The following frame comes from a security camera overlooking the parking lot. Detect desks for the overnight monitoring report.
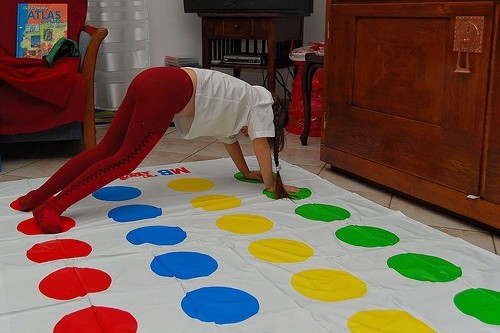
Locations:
[198,10,311,95]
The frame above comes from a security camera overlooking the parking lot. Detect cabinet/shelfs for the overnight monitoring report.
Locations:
[320,0,495,230]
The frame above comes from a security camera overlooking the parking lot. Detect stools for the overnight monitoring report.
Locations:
[299,52,324,146]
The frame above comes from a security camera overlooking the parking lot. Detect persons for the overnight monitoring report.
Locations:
[19,66,301,234]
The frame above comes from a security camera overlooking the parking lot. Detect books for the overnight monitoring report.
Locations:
[164,55,199,67]
[16,2,68,59]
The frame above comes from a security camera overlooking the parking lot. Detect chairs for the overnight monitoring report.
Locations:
[0,0,108,151]
